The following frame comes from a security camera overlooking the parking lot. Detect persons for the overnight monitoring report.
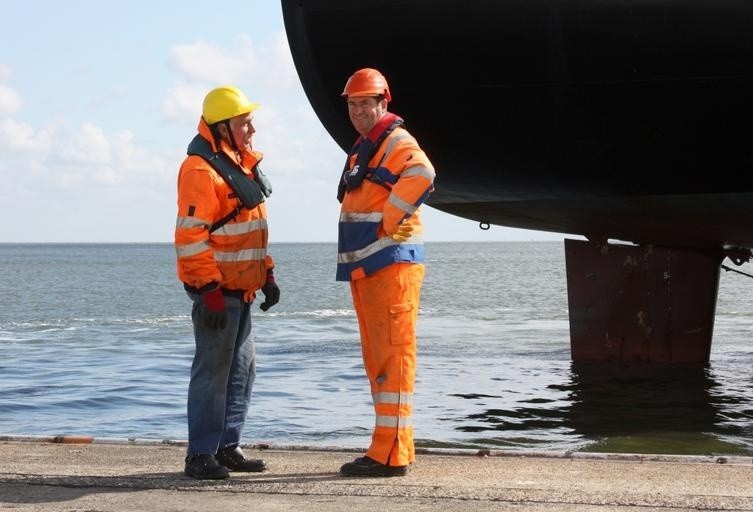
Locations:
[331,66,441,478]
[172,85,280,480]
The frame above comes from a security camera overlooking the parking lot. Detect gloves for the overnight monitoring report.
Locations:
[260,271,281,311]
[387,219,414,243]
[201,284,228,330]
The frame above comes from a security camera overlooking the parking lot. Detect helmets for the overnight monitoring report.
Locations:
[202,85,259,124]
[340,66,392,103]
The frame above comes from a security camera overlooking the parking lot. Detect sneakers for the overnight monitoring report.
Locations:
[354,455,413,472]
[340,463,408,476]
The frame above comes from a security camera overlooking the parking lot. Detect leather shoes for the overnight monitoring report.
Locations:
[216,447,269,473]
[185,454,230,481]
